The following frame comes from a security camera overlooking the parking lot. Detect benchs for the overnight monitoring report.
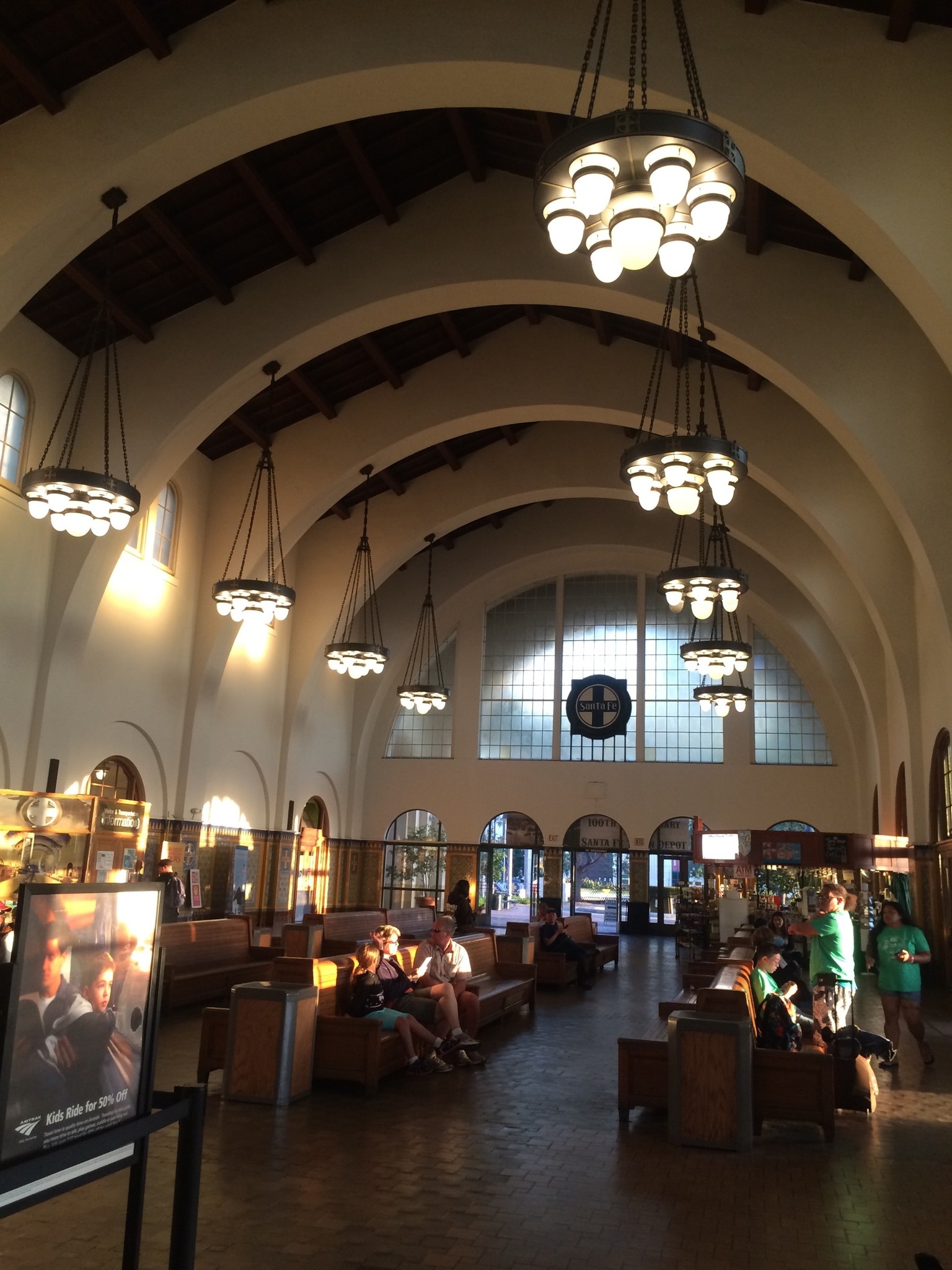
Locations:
[502,899,522,908]
[159,905,837,1146]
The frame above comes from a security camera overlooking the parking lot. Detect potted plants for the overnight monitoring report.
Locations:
[385,823,447,907]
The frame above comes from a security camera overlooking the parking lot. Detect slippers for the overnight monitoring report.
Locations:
[918,1041,934,1065]
[879,1060,899,1070]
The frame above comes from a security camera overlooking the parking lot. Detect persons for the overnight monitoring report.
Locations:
[4,921,137,1124]
[413,915,487,1068]
[541,907,593,989]
[343,942,461,1076]
[445,879,484,935]
[372,925,480,1067]
[750,911,815,1051]
[157,858,179,923]
[0,901,15,963]
[787,882,858,1032]
[865,901,935,1070]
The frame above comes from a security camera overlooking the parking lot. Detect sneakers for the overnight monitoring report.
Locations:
[404,1058,432,1074]
[467,1049,487,1065]
[425,1056,452,1072]
[435,1037,461,1058]
[445,1032,479,1045]
[455,1049,471,1066]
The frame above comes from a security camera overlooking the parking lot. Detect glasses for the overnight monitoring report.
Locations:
[430,927,447,933]
[816,894,844,902]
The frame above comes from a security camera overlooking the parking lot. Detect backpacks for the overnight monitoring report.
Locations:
[164,874,186,908]
[755,993,802,1052]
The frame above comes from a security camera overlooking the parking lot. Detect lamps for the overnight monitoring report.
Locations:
[22,186,146,538]
[190,808,201,819]
[656,493,749,621]
[679,600,753,681]
[396,534,451,715]
[532,0,747,285]
[94,764,110,780]
[211,360,296,626]
[693,672,753,717]
[324,462,389,679]
[619,264,750,517]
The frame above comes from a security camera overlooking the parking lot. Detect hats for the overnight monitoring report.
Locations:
[546,907,556,914]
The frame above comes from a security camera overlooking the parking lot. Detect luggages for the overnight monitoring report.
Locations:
[826,979,879,1119]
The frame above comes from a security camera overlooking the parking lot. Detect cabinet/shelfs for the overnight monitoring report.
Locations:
[675,897,711,964]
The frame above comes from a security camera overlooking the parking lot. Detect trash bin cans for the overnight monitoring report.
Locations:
[496,935,535,964]
[252,926,272,947]
[282,923,323,958]
[664,1008,753,1148]
[221,979,319,1107]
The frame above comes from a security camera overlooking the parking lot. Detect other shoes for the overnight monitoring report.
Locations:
[582,988,596,998]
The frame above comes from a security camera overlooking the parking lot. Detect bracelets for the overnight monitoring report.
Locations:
[910,954,914,963]
